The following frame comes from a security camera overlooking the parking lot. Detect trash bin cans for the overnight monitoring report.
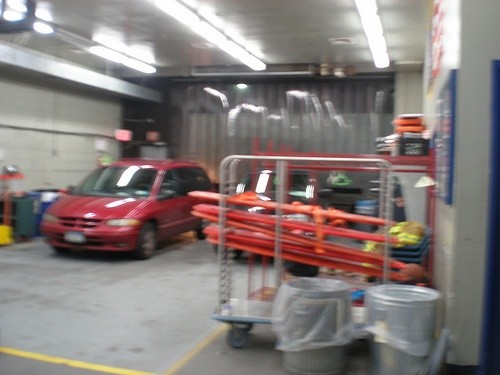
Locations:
[368,283,442,375]
[280,277,350,375]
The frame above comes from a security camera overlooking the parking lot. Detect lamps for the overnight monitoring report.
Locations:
[355,0,391,69]
[149,0,267,71]
[87,44,156,75]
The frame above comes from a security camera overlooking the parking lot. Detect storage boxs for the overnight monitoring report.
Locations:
[395,137,431,156]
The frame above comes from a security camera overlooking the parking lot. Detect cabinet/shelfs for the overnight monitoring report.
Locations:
[0,196,35,237]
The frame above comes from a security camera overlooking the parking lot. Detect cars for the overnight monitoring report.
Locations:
[39,158,219,260]
[212,167,338,262]
